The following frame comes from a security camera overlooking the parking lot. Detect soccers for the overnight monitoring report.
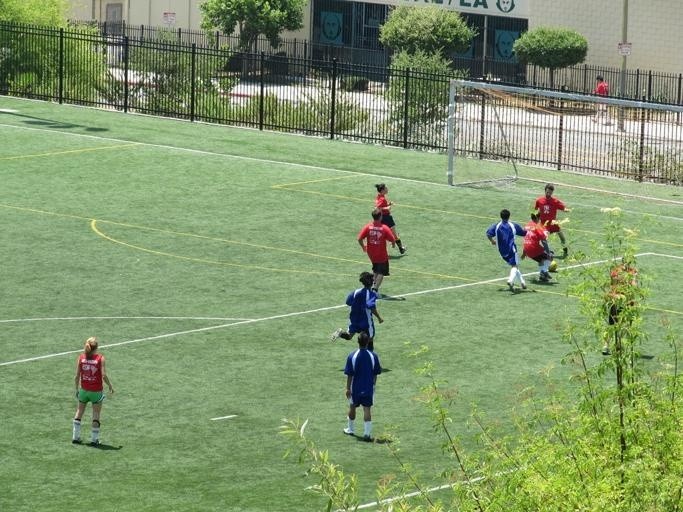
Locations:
[548,261,557,272]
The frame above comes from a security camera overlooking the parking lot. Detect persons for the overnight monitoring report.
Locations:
[592,75,611,125]
[602,258,639,356]
[344,333,381,441]
[72,338,115,447]
[330,273,384,352]
[375,184,407,255]
[486,210,527,292]
[520,211,554,282]
[358,208,396,298]
[535,184,571,257]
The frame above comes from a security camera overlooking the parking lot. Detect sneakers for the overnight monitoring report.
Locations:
[329,328,343,344]
[71,437,102,446]
[505,270,553,291]
[600,346,613,356]
[371,287,381,299]
[398,244,408,255]
[341,427,374,441]
